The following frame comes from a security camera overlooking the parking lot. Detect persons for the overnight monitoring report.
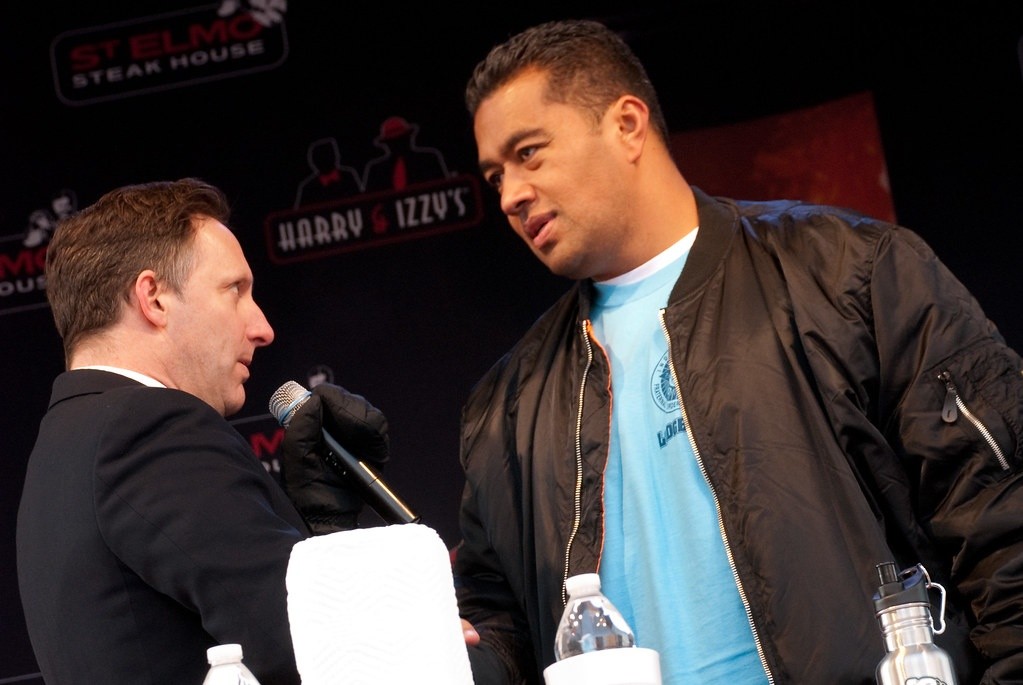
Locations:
[16,176,479,685]
[451,22,1023,685]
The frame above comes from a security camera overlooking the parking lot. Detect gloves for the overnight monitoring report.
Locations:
[279,383,393,538]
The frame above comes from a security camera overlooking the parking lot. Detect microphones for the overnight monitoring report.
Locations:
[269,381,424,525]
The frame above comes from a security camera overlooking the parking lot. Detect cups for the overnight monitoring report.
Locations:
[542,646,663,685]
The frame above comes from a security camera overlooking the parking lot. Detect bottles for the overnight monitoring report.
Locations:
[554,573,635,663]
[201,643,261,685]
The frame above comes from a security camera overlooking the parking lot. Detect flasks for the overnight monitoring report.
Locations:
[872,560,958,685]
